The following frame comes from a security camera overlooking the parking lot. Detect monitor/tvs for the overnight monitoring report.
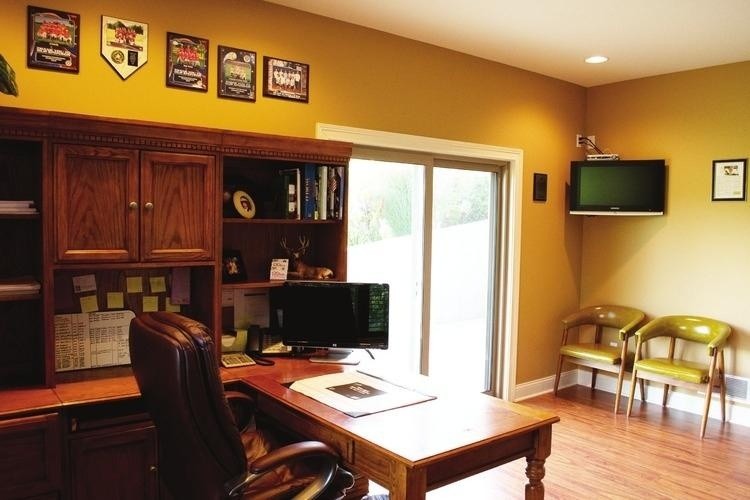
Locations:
[568,160,665,216]
[269,283,389,364]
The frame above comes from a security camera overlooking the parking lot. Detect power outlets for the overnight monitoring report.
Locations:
[586,135,595,149]
[576,134,583,148]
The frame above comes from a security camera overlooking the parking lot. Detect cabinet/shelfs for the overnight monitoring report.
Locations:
[0,105,358,499]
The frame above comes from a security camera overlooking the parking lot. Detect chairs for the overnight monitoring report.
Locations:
[553,304,647,413]
[626,315,732,439]
[128,310,354,500]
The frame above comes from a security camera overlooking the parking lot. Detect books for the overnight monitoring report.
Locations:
[279,162,346,222]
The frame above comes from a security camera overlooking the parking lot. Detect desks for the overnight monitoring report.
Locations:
[242,362,560,499]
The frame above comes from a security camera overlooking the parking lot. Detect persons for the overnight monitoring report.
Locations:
[241,197,251,212]
[175,43,202,70]
[229,64,248,82]
[114,24,137,46]
[37,19,73,44]
[275,66,300,91]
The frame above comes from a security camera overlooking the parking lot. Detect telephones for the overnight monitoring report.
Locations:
[248,324,293,353]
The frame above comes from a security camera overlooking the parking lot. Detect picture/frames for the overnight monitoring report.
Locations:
[712,159,747,201]
[101,15,148,80]
[217,44,256,102]
[26,5,80,74]
[263,55,310,104]
[165,31,209,93]
